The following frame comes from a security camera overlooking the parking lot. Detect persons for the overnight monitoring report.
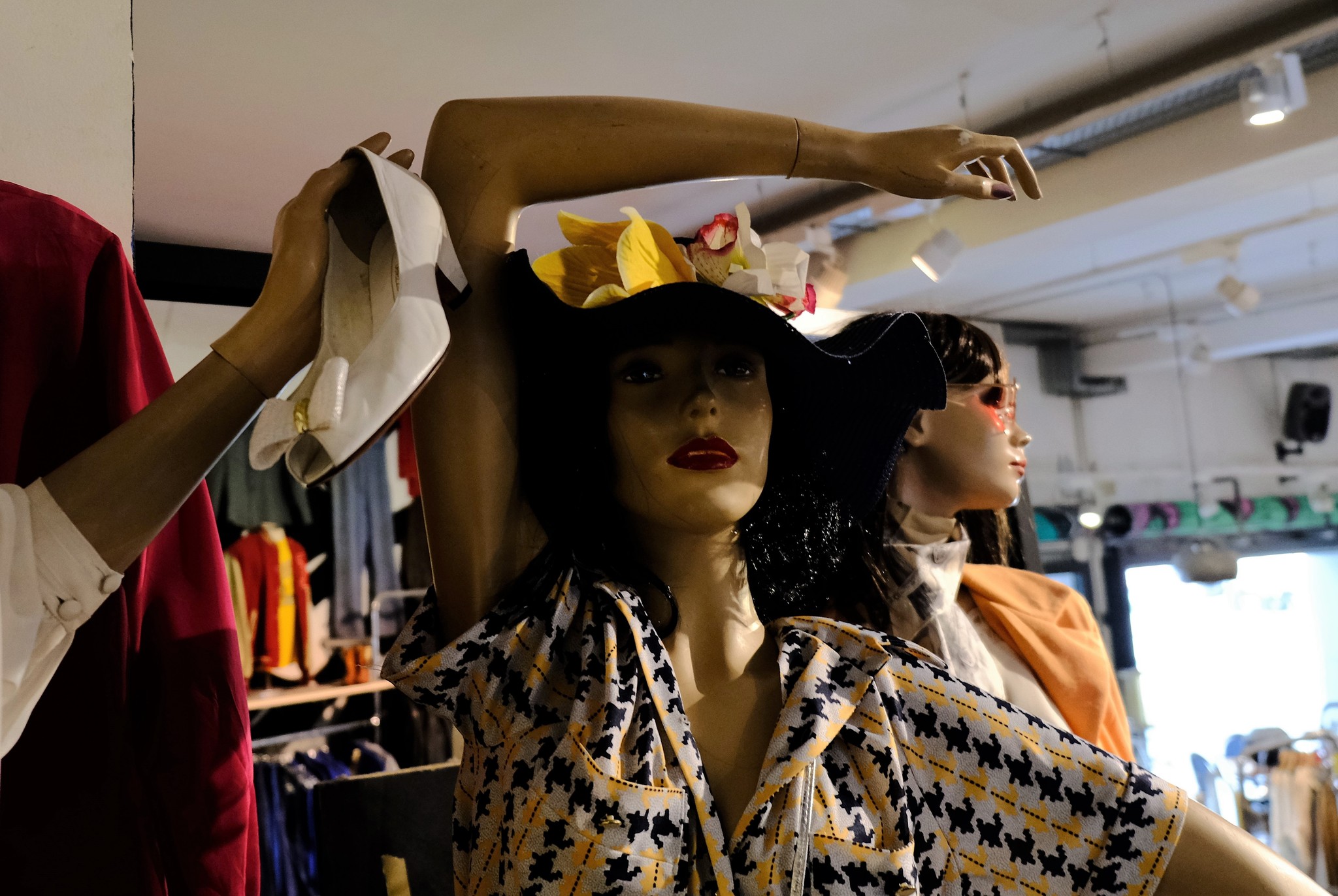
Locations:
[0,130,410,765]
[375,96,1332,896]
[812,310,1137,767]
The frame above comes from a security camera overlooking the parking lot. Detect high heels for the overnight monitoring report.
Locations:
[251,148,471,491]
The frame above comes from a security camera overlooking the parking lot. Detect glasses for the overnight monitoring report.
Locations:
[948,383,1017,431]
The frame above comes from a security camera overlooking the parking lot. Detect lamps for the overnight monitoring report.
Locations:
[1233,59,1303,146]
[1074,492,1109,537]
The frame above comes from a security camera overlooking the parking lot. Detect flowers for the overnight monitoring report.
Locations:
[534,203,828,327]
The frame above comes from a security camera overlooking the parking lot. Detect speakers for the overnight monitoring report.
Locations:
[1284,382,1331,442]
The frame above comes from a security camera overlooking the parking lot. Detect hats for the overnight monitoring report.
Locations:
[509,241,950,617]
